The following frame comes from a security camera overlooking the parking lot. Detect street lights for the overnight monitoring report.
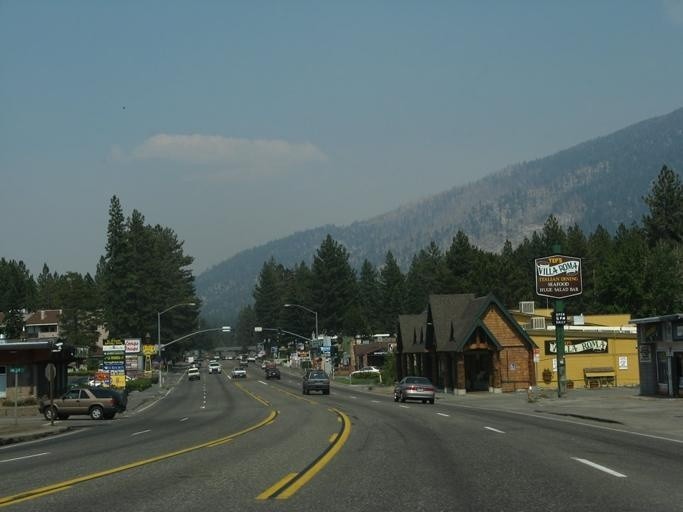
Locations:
[158,303,196,387]
[284,304,318,338]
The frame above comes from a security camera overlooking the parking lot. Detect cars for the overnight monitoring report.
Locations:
[262,361,280,380]
[352,366,379,375]
[231,367,246,378]
[394,376,435,404]
[303,370,329,395]
[187,362,201,380]
[40,388,127,419]
[240,358,255,367]
[208,360,223,374]
